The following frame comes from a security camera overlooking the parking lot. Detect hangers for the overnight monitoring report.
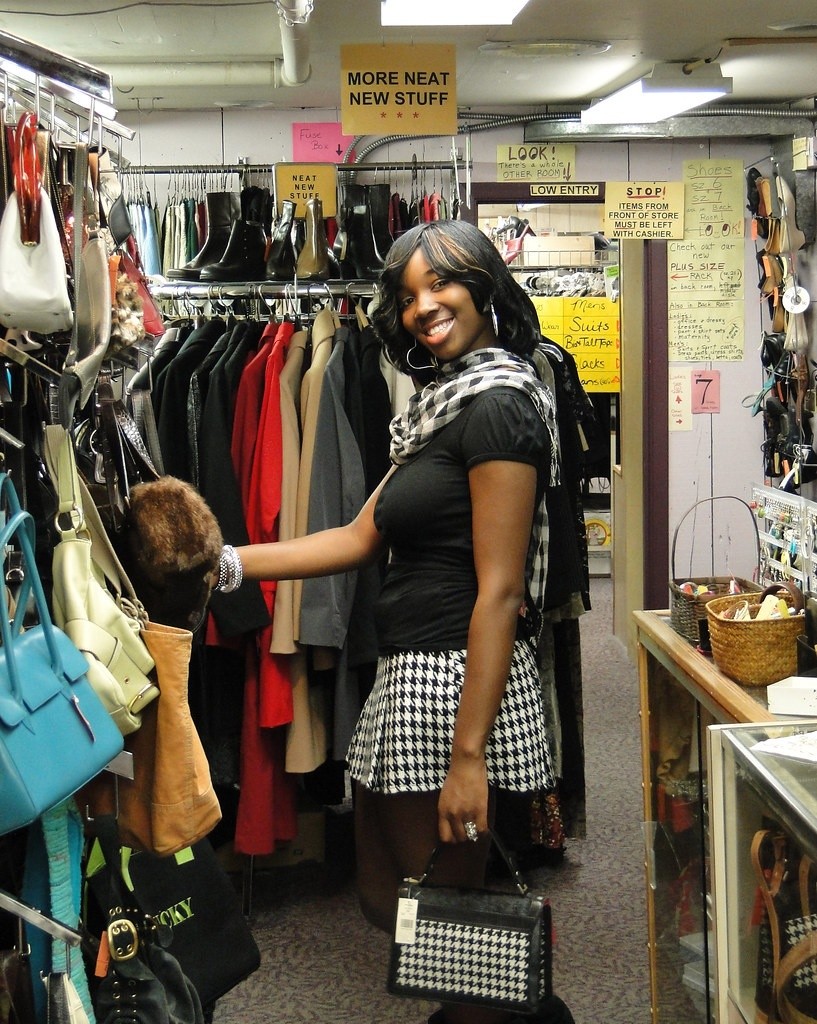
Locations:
[523,272,607,298]
[112,160,457,212]
[152,283,383,334]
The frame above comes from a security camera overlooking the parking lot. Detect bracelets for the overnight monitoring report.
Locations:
[212,545,242,593]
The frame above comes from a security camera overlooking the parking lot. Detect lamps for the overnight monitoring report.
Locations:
[579,59,732,125]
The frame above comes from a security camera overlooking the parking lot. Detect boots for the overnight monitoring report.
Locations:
[167,183,401,283]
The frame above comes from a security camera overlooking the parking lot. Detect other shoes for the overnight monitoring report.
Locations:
[743,166,817,498]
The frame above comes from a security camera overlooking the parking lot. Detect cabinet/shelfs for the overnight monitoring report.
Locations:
[633,609,817,1023]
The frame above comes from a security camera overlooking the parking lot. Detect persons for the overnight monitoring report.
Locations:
[211,219,573,1024]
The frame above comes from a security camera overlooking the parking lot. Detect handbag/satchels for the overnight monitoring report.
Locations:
[0,101,261,1024]
[748,810,817,1024]
[384,826,553,1014]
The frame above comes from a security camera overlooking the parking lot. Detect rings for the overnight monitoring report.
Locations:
[463,822,478,842]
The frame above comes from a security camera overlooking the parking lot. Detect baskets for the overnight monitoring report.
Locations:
[668,495,761,645]
[704,592,806,687]
